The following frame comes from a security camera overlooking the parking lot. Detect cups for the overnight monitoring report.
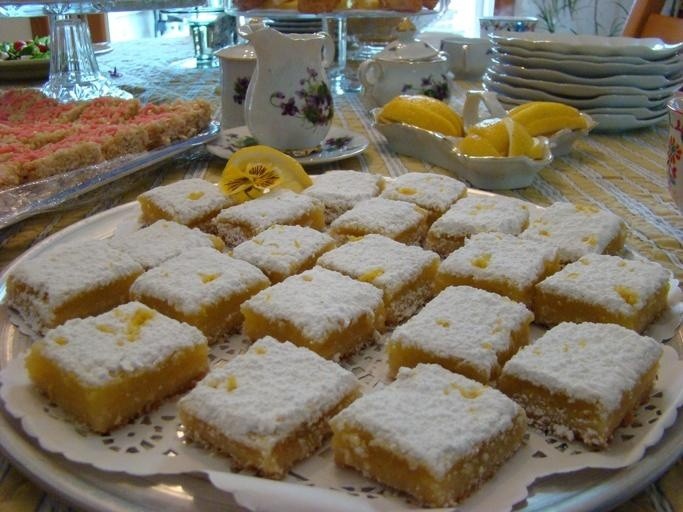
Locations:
[215,41,257,119]
[442,37,490,77]
[479,17,538,40]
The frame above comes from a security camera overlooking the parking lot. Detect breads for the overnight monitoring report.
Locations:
[519,201,625,261]
[110,217,215,267]
[329,362,528,505]
[379,171,467,217]
[498,322,663,448]
[329,197,432,247]
[136,176,228,228]
[176,336,364,480]
[317,233,441,330]
[228,222,336,284]
[533,251,671,338]
[240,265,388,362]
[6,237,145,335]
[129,243,272,346]
[433,231,560,304]
[384,285,533,385]
[25,300,211,434]
[423,196,530,257]
[215,190,325,245]
[1,88,210,194]
[299,170,386,225]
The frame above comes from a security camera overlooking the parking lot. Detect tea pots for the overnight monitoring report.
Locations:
[358,17,452,105]
[237,24,334,151]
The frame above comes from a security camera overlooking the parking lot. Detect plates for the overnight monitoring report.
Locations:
[482,83,670,118]
[490,40,683,64]
[491,48,683,78]
[483,72,683,109]
[487,32,683,61]
[269,22,322,27]
[371,108,594,189]
[501,102,670,120]
[277,27,322,33]
[0,41,113,74]
[590,113,669,131]
[0,184,683,512]
[488,67,683,100]
[265,13,323,21]
[205,125,370,164]
[492,58,683,89]
